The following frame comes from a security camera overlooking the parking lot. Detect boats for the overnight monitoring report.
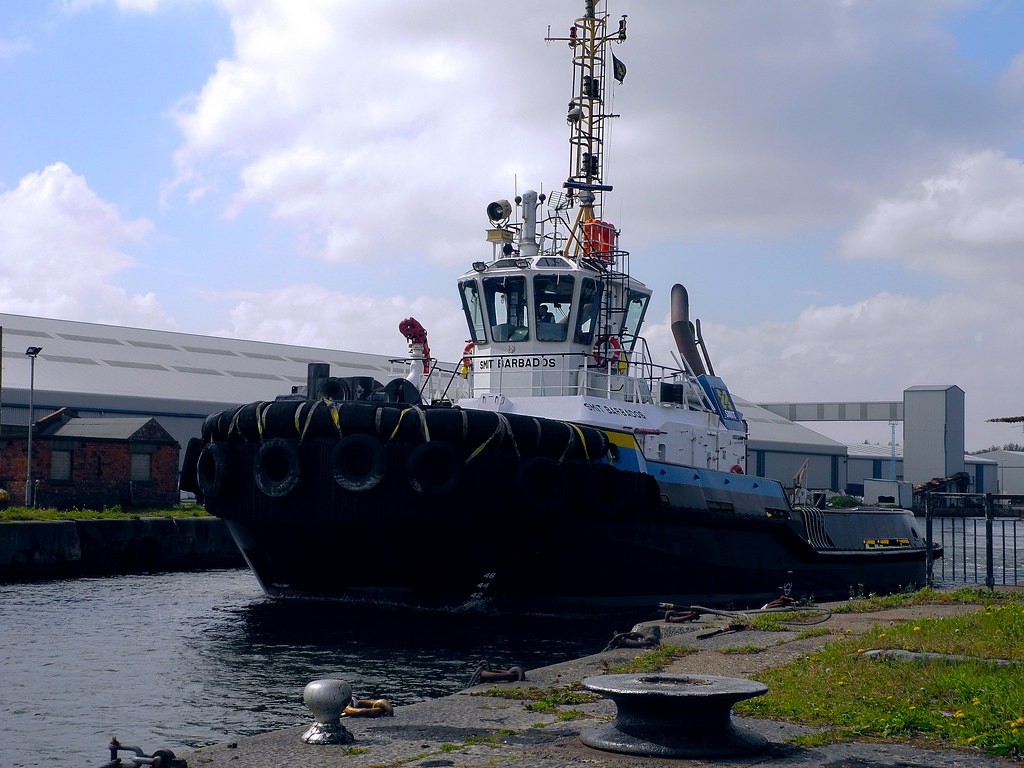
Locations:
[178,2,825,637]
[791,504,943,599]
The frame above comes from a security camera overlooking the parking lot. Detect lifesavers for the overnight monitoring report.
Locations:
[462,343,474,364]
[516,454,566,512]
[330,434,388,493]
[592,336,620,366]
[582,462,628,520]
[195,443,231,499]
[251,438,300,498]
[406,441,462,497]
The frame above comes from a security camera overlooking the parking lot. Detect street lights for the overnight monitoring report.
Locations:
[25,347,42,507]
[999,459,1007,507]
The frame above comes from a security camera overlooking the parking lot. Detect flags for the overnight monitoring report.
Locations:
[612,53,626,84]
[794,458,807,486]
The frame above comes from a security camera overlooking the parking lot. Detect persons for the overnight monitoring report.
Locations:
[538,304,555,322]
